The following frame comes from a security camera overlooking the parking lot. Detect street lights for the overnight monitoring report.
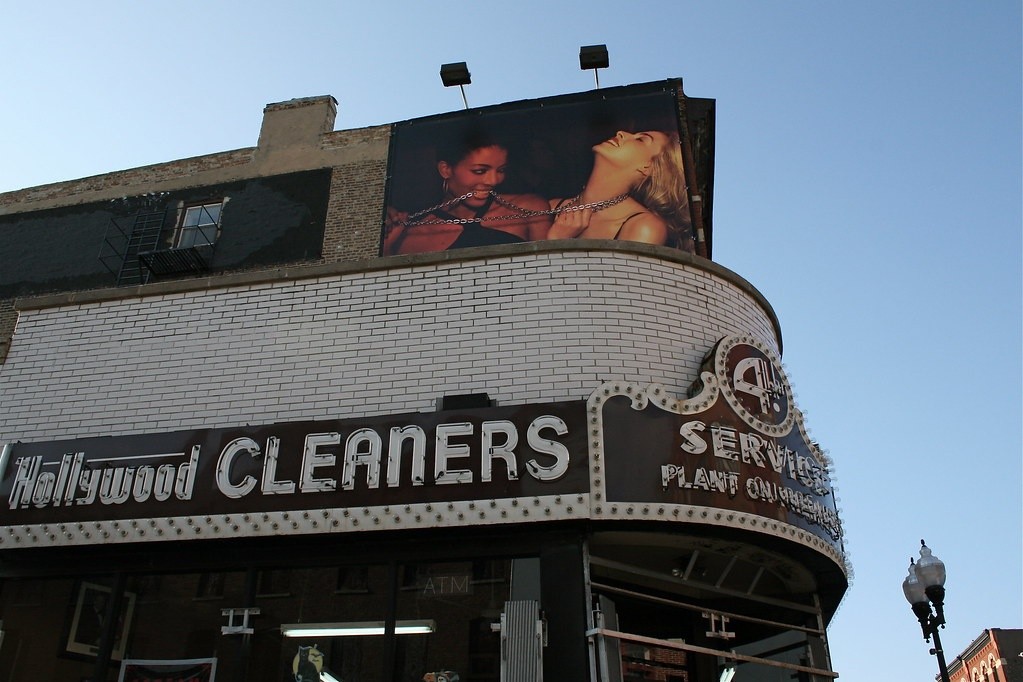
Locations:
[902,539,951,682]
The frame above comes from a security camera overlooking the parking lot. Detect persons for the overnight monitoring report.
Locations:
[548,131,694,253]
[384,135,552,255]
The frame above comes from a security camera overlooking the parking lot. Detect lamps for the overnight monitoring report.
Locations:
[580,43,610,89]
[279,614,441,639]
[439,61,472,110]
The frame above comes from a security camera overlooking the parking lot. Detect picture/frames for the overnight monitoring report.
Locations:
[58,579,141,664]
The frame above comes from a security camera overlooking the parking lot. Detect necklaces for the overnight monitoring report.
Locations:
[386,190,631,225]
